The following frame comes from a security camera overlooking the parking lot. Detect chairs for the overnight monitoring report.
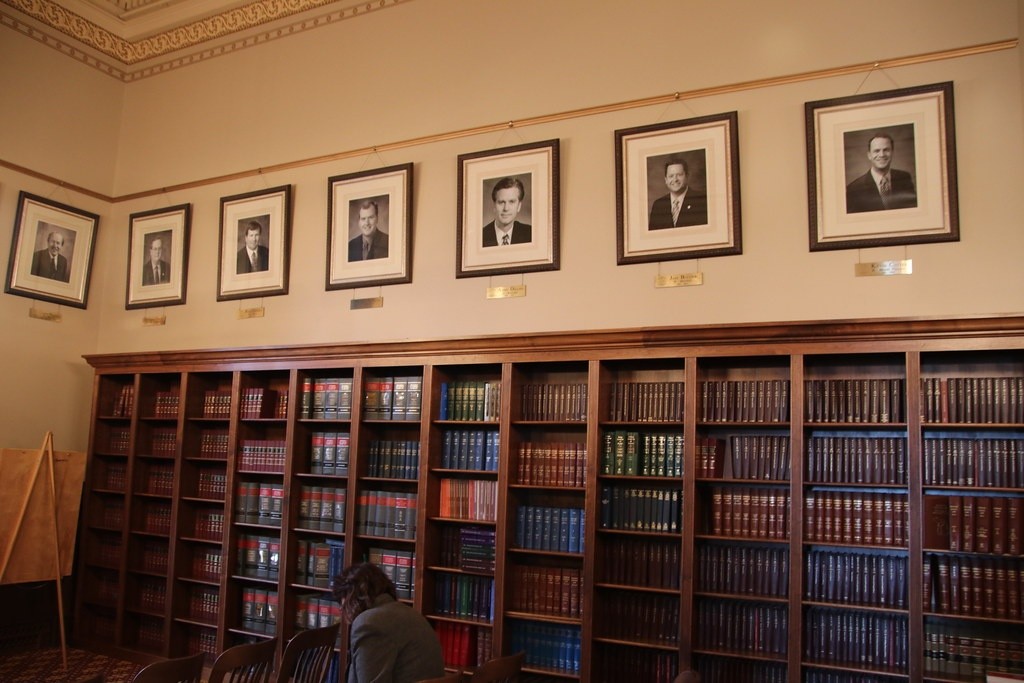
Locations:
[126,621,525,683]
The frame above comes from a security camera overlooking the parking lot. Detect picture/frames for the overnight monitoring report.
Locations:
[455,139,561,279]
[614,111,743,266]
[805,80,960,251]
[4,190,101,311]
[216,183,292,302]
[325,162,413,293]
[125,202,190,310]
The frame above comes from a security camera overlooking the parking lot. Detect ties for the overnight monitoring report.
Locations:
[672,199,679,226]
[51,257,55,274]
[364,240,369,251]
[502,234,510,245]
[880,177,890,210]
[251,252,256,265]
[154,265,160,284]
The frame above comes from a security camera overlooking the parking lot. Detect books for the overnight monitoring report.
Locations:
[84,378,1024,683]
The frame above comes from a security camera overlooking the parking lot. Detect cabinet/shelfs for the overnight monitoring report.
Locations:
[61,315,1024,683]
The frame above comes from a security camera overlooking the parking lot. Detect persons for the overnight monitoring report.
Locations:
[142,237,170,286]
[237,221,269,274]
[31,232,68,282]
[846,133,917,214]
[333,563,446,683]
[482,177,532,247]
[348,201,389,263]
[649,159,708,231]
